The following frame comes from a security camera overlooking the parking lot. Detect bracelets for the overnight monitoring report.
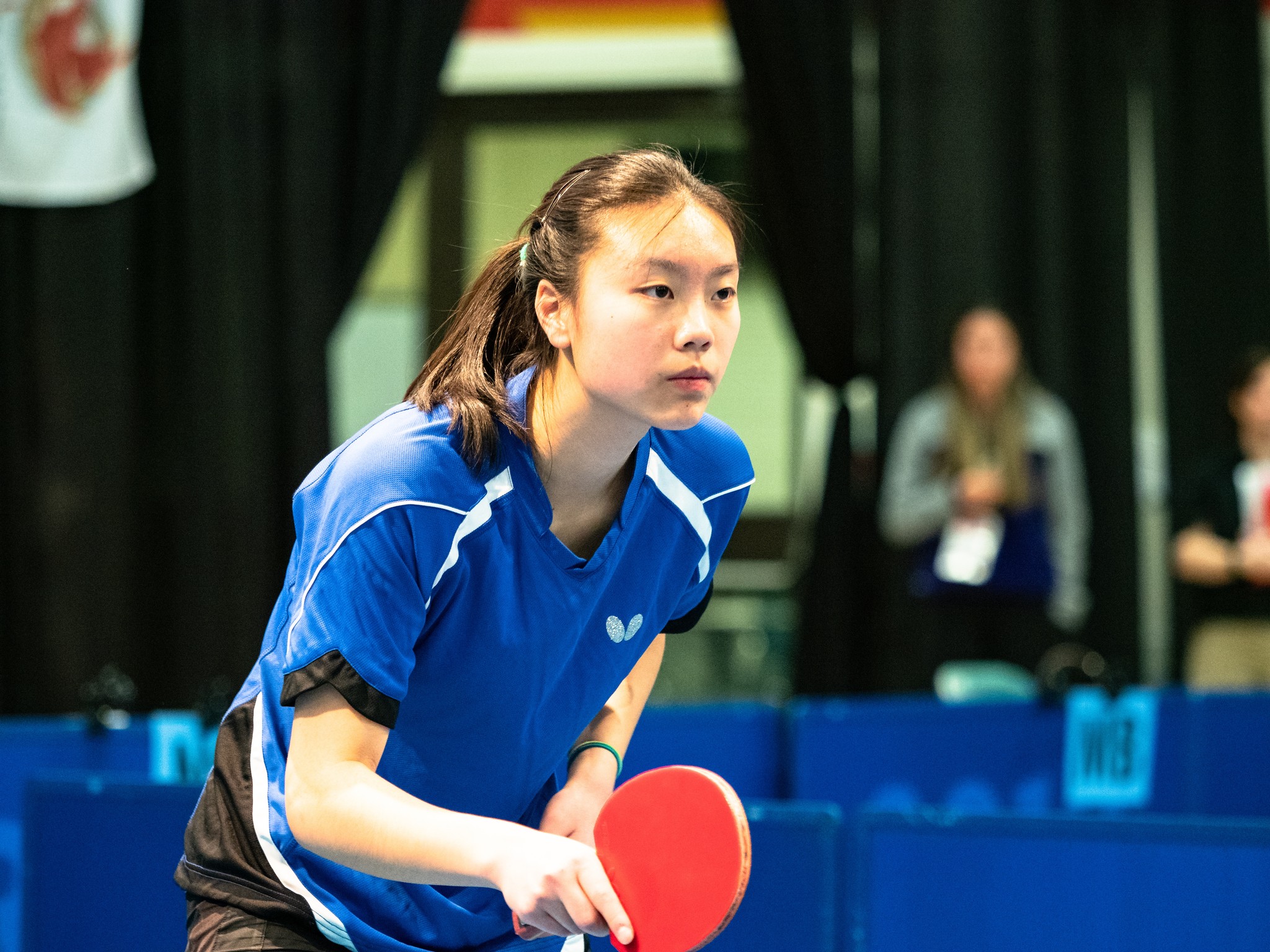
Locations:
[567,741,623,778]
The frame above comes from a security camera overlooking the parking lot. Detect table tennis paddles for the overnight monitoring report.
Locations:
[511,763,753,952]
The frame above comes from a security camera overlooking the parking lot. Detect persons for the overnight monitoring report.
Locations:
[1171,337,1270,685]
[174,137,757,952]
[880,294,1090,630]
